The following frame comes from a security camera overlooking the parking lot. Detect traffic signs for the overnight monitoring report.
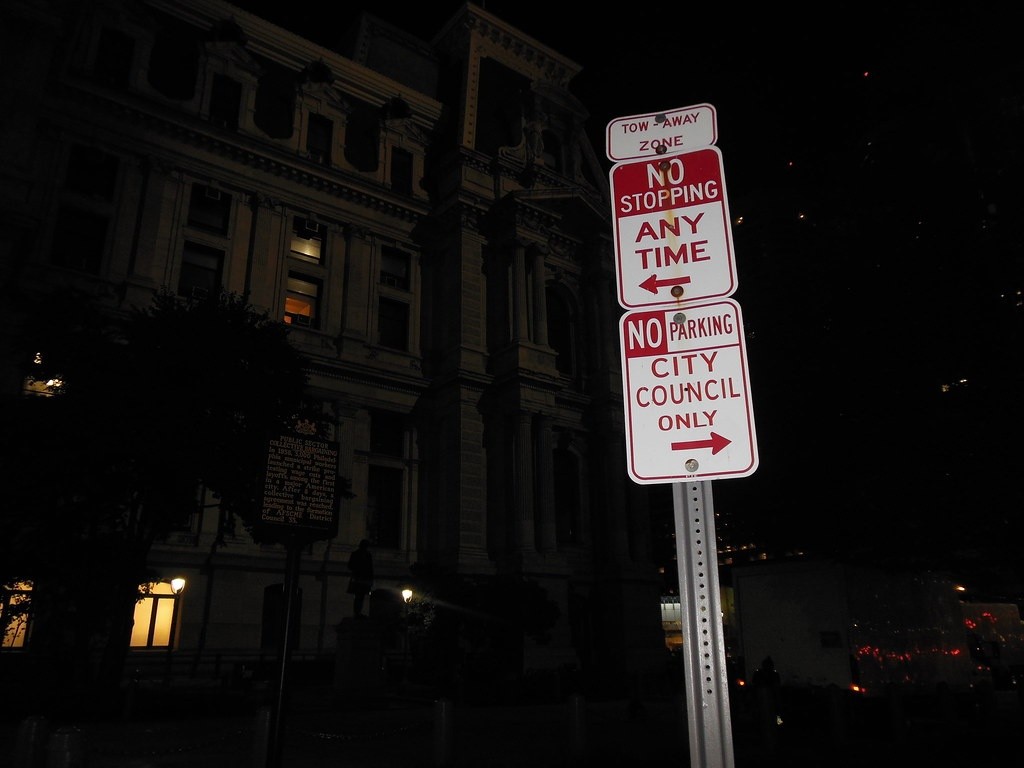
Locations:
[611,145,737,312]
[619,300,760,488]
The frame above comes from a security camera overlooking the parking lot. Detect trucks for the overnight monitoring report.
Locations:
[729,555,1008,736]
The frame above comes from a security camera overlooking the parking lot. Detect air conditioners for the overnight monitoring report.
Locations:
[291,314,311,328]
[302,220,319,237]
[191,286,209,301]
[204,186,221,206]
